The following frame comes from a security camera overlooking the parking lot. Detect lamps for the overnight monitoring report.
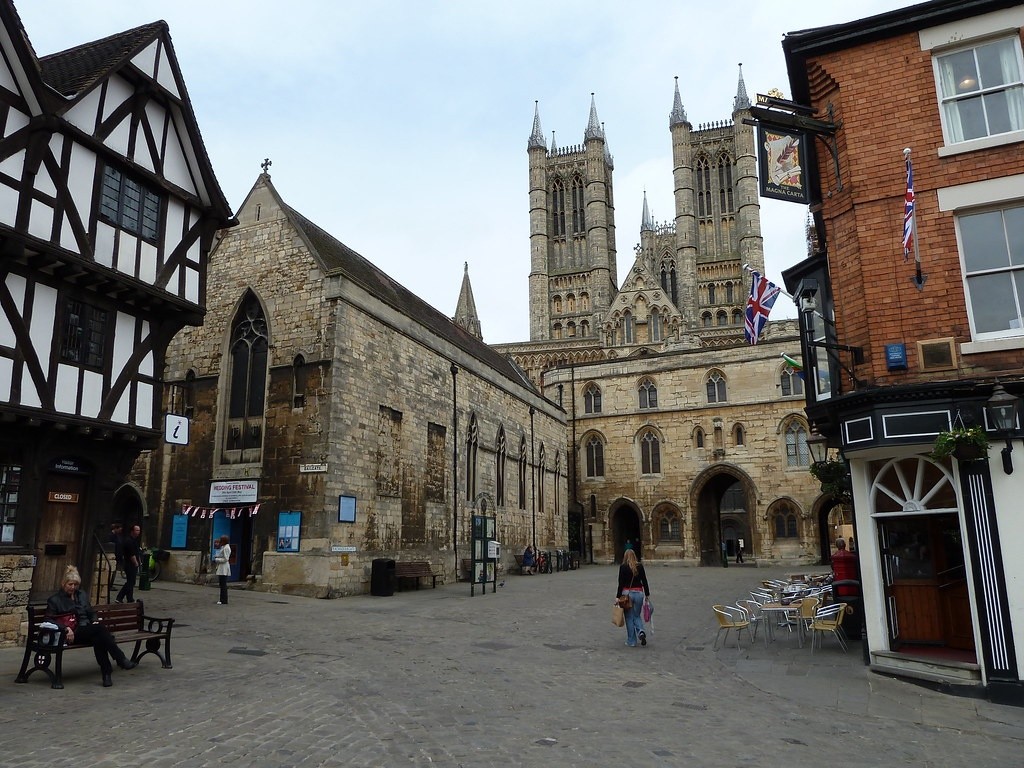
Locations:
[804,421,832,463]
[99,428,114,438]
[958,73,976,90]
[985,375,1020,474]
[79,426,92,434]
[27,417,43,426]
[0,412,18,422]
[54,423,67,429]
[123,432,138,441]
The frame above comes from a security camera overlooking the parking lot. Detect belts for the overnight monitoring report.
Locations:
[624,588,642,590]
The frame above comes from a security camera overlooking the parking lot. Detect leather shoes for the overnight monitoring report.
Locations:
[102,675,112,687]
[117,657,137,670]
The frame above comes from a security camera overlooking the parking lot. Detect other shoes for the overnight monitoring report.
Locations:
[625,642,636,646]
[639,633,646,646]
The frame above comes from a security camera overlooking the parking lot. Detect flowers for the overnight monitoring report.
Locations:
[807,457,844,473]
[930,424,990,462]
[821,478,850,499]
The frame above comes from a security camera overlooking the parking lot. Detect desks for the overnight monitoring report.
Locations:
[760,603,806,649]
[787,572,832,582]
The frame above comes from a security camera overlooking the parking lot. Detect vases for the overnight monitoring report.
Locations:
[951,436,980,461]
[816,473,842,483]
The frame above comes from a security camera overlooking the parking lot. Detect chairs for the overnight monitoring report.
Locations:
[713,570,849,654]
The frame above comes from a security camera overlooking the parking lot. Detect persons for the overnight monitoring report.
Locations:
[623,538,641,562]
[110,524,122,592]
[835,535,854,551]
[114,525,142,604]
[722,540,728,568]
[43,564,135,687]
[522,545,537,575]
[614,549,651,647]
[213,535,232,606]
[736,539,744,563]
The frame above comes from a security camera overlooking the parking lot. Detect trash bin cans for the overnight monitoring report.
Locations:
[831,580,863,641]
[371,557,396,596]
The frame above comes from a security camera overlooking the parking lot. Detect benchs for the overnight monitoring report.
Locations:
[394,561,444,590]
[462,557,501,582]
[514,554,539,575]
[14,598,176,689]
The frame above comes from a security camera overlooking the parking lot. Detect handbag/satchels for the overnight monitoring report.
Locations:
[37,621,61,646]
[643,601,653,622]
[53,612,77,629]
[611,604,624,627]
[619,595,632,608]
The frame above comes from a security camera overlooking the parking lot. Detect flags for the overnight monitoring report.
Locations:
[744,268,780,346]
[901,158,914,262]
